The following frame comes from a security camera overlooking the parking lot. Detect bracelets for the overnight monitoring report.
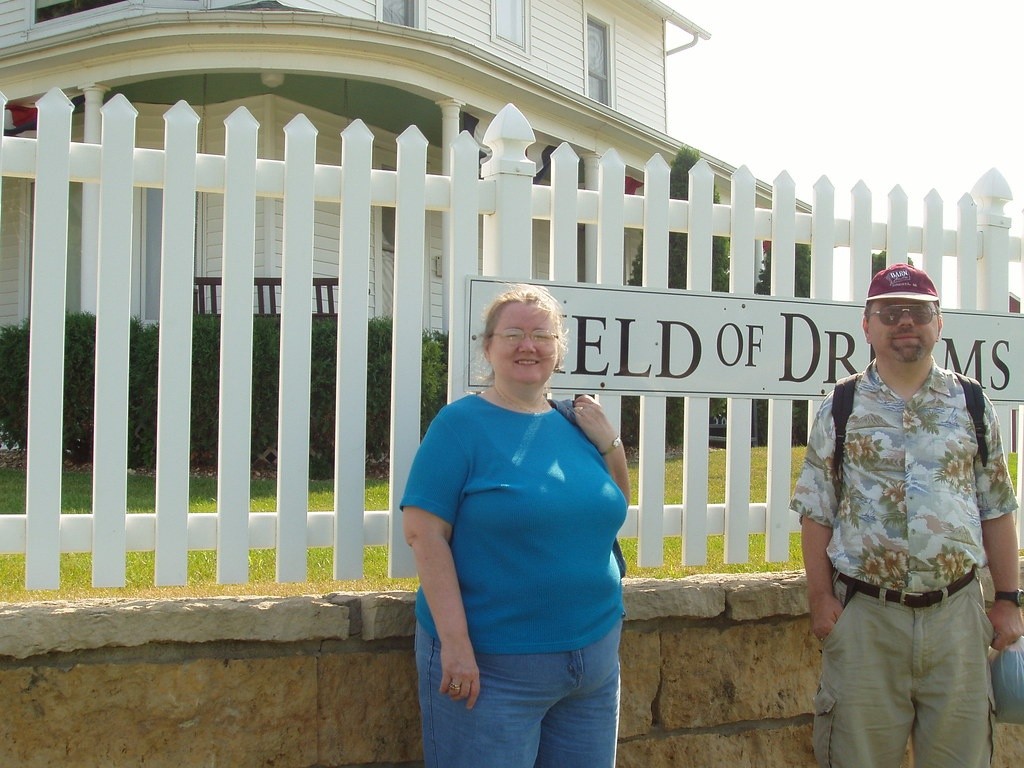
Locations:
[601,437,621,455]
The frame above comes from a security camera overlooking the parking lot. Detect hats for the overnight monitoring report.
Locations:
[866,263,939,300]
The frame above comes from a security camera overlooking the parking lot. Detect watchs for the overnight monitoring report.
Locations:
[995,589,1024,607]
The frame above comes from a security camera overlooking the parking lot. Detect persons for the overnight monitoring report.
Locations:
[400,286,631,768]
[788,264,1024,767]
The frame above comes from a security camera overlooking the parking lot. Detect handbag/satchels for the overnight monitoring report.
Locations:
[988,636,1024,725]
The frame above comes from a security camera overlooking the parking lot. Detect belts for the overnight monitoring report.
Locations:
[838,567,976,608]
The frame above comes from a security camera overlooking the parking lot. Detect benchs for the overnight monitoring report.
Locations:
[194,276,371,320]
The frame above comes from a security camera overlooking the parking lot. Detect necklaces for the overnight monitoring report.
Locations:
[495,387,545,413]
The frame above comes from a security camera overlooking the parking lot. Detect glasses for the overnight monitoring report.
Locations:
[488,328,561,347]
[866,305,938,326]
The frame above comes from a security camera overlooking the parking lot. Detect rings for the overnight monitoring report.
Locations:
[577,407,583,413]
[820,638,823,641]
[448,683,461,690]
